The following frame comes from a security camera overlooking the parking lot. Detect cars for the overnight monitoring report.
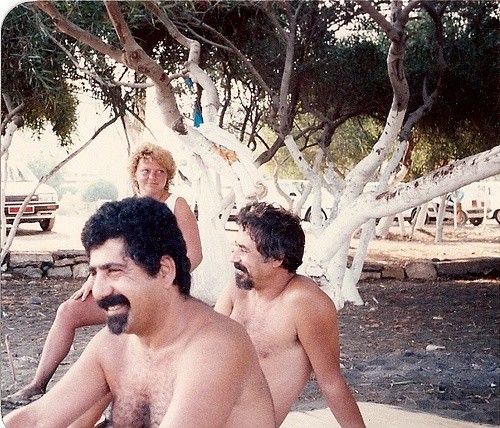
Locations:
[6,161,59,234]
[199,173,499,227]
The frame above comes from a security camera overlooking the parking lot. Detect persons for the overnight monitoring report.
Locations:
[2,198,276,427]
[3,144,205,401]
[212,200,370,428]
